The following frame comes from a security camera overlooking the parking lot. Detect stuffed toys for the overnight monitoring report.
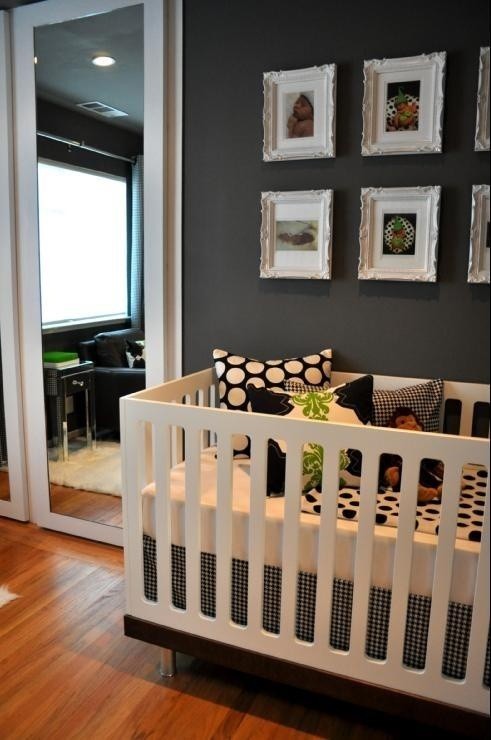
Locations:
[379,404,466,501]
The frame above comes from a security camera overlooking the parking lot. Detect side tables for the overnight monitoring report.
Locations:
[39,358,96,457]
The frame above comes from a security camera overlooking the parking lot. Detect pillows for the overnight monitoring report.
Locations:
[214,347,334,461]
[366,380,444,473]
[245,376,381,496]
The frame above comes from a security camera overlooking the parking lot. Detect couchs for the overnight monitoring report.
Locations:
[80,328,150,433]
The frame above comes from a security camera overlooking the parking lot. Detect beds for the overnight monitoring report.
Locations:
[121,354,491,740]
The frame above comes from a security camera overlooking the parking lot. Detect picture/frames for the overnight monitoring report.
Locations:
[475,44,491,152]
[359,187,440,283]
[363,52,444,157]
[260,190,333,281]
[263,63,337,160]
[467,183,491,284]
